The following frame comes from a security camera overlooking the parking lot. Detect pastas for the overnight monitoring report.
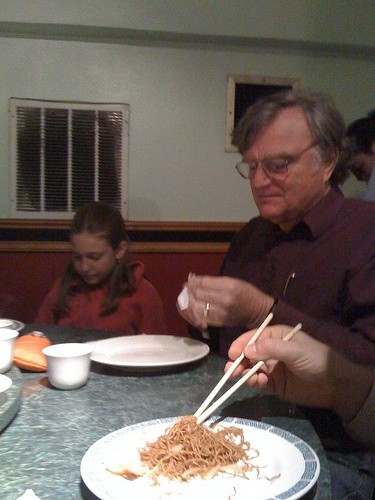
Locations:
[106,416,280,487]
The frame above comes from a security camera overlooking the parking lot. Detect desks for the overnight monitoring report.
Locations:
[1,324,332,499]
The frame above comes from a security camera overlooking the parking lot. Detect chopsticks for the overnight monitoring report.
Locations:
[193,311,303,424]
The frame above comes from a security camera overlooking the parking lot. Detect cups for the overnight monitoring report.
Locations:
[42,343,92,389]
[0,319,20,374]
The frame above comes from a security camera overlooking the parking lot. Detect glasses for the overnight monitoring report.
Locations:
[234,137,326,180]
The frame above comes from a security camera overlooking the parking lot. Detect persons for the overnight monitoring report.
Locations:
[223,321,375,451]
[33,201,166,334]
[178,84,375,366]
[343,110,375,201]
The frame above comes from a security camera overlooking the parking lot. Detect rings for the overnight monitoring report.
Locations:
[204,302,211,318]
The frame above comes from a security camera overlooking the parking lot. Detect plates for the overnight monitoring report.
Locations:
[80,414,320,499]
[0,374,12,394]
[87,333,210,367]
